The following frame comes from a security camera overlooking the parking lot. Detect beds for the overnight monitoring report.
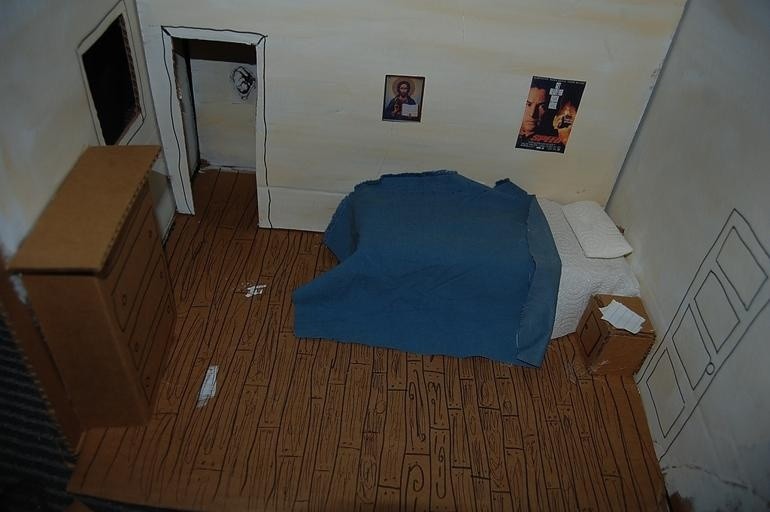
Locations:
[355,179,639,342]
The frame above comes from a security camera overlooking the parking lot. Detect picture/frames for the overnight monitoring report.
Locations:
[383,75,425,122]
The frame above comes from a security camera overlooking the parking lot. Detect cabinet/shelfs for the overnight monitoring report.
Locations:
[21,182,176,427]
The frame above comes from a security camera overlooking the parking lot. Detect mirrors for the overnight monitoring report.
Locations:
[75,0,147,148]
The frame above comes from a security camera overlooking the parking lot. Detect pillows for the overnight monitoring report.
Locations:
[563,202,634,259]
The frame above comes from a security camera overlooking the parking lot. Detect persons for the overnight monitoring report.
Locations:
[384,82,417,119]
[518,84,551,139]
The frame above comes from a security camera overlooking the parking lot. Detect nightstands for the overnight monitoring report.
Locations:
[575,294,654,376]
